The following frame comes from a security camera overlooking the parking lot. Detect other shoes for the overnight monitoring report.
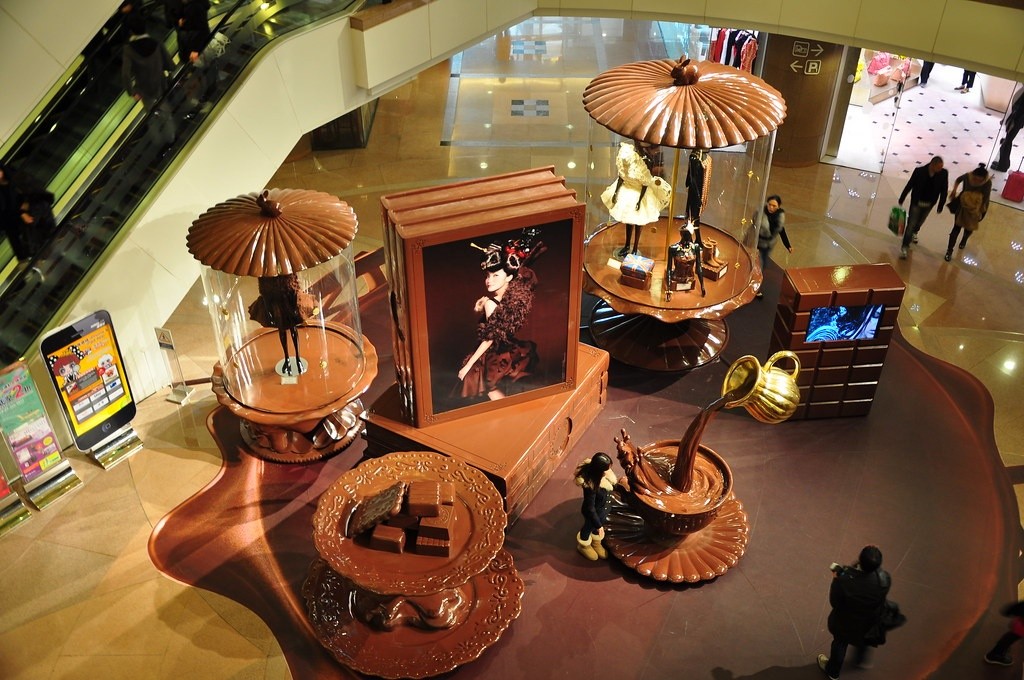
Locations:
[986,649,1013,665]
[960,88,969,93]
[954,86,965,90]
[920,83,926,88]
[945,249,952,261]
[899,247,908,259]
[757,292,763,298]
[960,240,965,249]
[913,233,918,243]
[817,654,839,680]
[991,161,1007,172]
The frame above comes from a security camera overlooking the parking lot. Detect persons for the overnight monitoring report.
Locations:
[572,453,618,562]
[600,138,670,256]
[0,160,65,296]
[919,61,934,88]
[121,0,217,157]
[743,194,792,299]
[806,305,882,341]
[247,273,318,372]
[817,545,893,680]
[991,92,1024,172]
[944,163,993,261]
[685,148,712,248]
[954,69,976,94]
[449,226,546,401]
[664,223,707,302]
[898,156,949,259]
[983,579,1024,666]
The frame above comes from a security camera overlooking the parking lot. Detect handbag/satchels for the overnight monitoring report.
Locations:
[946,196,959,214]
[867,51,891,74]
[874,65,892,87]
[901,59,911,78]
[888,204,906,237]
[890,64,905,82]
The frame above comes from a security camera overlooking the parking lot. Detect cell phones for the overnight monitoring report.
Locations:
[39,309,138,453]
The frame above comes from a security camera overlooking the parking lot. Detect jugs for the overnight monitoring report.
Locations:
[720,350,801,425]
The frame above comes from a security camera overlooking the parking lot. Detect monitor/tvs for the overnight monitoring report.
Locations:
[804,304,884,342]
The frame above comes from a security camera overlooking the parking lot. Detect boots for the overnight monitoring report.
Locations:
[576,531,598,561]
[592,530,607,560]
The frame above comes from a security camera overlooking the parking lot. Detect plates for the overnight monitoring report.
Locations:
[301,543,524,680]
[308,449,508,595]
[606,473,748,585]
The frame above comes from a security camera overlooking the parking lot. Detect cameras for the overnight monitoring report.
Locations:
[829,563,846,576]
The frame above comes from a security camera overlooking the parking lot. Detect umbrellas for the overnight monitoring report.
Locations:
[186,187,358,277]
[583,56,786,260]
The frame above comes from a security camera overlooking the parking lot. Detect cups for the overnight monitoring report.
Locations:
[624,441,734,539]
